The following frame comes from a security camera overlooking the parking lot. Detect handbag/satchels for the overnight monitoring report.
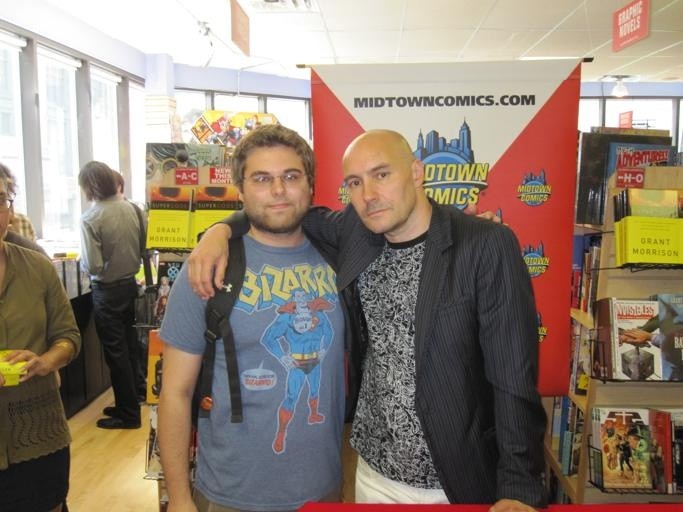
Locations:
[134,292,156,325]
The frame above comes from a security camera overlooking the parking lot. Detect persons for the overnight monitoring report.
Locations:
[3,230,52,262]
[111,167,147,405]
[155,122,347,512]
[186,128,548,512]
[8,174,36,241]
[0,161,82,511]
[79,161,143,430]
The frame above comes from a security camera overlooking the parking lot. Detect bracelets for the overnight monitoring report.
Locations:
[50,341,76,361]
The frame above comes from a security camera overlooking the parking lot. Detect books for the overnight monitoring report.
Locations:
[145,140,238,512]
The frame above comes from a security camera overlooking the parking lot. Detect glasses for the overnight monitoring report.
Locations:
[242,171,303,185]
[1,198,15,211]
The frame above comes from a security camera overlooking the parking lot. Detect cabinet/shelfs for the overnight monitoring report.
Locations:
[57,293,111,420]
[142,190,244,512]
[541,127,683,512]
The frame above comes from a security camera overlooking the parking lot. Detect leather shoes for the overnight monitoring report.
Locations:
[88,278,136,290]
[96,404,141,430]
